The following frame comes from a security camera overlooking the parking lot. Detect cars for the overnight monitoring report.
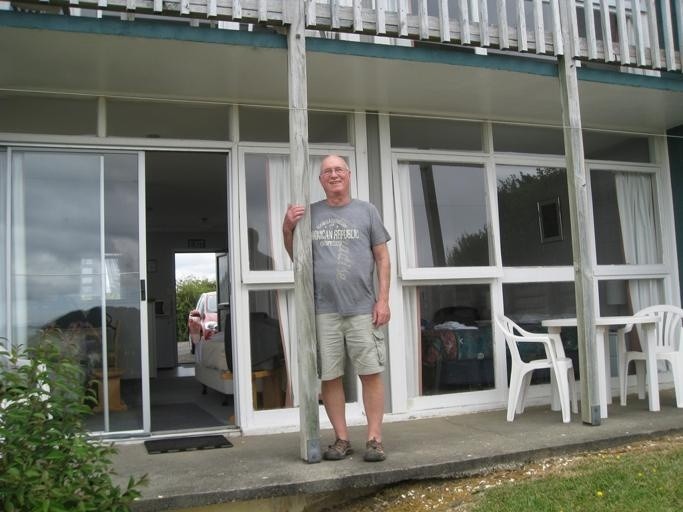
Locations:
[186,290,218,354]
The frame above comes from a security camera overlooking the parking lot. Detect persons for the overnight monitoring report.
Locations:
[282,153,393,462]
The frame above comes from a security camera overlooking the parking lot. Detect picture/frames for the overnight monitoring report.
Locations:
[536,195,563,244]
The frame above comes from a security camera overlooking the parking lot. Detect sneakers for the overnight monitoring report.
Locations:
[323,438,352,460]
[363,438,386,462]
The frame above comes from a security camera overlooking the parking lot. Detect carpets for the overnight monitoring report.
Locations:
[133,401,233,455]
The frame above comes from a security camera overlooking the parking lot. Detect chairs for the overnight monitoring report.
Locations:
[493,304,682,425]
